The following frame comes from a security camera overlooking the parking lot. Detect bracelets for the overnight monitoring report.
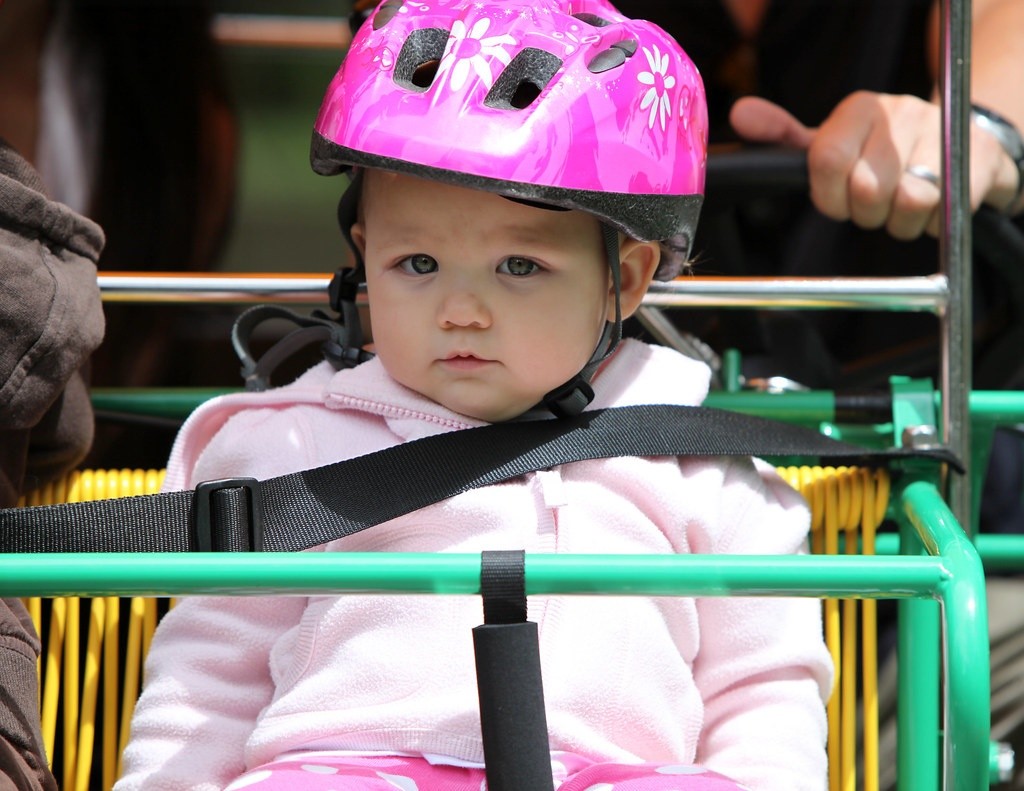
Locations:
[970,104,1024,216]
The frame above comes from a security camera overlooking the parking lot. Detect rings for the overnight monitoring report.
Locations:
[906,166,941,188]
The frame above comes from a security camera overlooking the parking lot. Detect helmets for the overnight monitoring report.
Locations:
[307,0,710,283]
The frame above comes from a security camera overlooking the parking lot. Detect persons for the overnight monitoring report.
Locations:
[111,0,835,791]
[610,0,1024,241]
